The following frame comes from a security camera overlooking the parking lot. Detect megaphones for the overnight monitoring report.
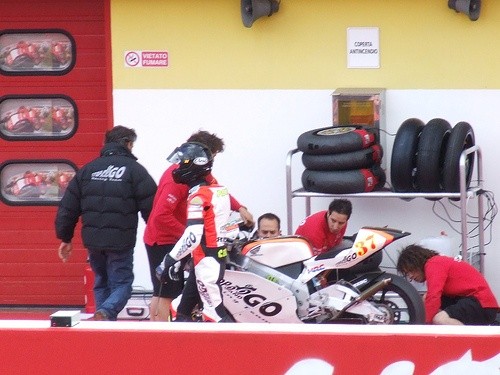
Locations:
[241,0,279,28]
[448,0,481,21]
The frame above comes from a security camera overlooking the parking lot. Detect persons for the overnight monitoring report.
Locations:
[155,142,237,323]
[144,131,254,321]
[257,213,281,238]
[295,199,352,256]
[55,125,158,320]
[396,245,500,326]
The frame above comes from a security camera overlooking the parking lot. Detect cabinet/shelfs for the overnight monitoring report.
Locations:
[286,145,487,299]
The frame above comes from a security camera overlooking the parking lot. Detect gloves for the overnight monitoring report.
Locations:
[155,253,178,282]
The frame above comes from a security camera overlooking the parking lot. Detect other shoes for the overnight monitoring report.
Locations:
[94,309,116,321]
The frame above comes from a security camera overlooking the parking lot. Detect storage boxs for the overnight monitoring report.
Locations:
[331,84,387,135]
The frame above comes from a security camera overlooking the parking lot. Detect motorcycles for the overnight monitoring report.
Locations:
[167,218,427,324]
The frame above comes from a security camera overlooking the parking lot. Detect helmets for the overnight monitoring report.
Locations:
[172,143,213,184]
